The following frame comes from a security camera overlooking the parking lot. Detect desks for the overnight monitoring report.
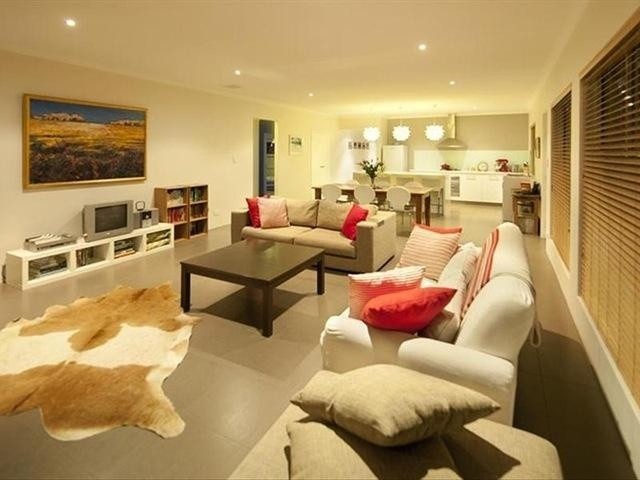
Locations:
[180,238,326,338]
[311,185,434,226]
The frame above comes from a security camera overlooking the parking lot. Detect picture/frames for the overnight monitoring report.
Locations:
[288,135,304,156]
[22,93,149,193]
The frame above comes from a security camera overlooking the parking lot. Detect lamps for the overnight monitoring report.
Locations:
[392,121,412,142]
[363,127,381,142]
[425,116,445,142]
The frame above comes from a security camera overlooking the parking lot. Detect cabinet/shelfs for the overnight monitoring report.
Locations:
[153,183,209,244]
[445,170,527,204]
[6,222,175,291]
[512,190,541,237]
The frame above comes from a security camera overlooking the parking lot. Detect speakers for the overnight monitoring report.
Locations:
[135,207,160,228]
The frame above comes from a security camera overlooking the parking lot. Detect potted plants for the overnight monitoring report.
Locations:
[355,157,384,189]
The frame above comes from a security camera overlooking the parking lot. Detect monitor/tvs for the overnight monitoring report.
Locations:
[82,199,134,243]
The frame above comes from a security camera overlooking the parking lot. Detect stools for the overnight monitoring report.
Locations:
[227,370,564,480]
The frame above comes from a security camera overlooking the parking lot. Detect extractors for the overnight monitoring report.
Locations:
[437,113,467,150]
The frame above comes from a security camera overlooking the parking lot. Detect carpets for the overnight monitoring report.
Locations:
[1,280,202,443]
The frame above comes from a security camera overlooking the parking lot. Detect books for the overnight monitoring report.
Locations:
[167,187,208,236]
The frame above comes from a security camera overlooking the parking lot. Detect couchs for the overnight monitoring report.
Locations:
[231,210,397,272]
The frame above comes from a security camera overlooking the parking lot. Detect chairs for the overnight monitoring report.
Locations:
[386,186,414,232]
[354,185,378,205]
[322,184,342,202]
[430,187,444,217]
[404,182,423,187]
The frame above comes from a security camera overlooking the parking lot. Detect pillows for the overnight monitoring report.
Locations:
[257,197,290,228]
[397,226,462,282]
[415,222,462,254]
[285,199,319,228]
[317,199,355,231]
[347,266,426,319]
[246,198,260,228]
[291,363,502,447]
[439,241,483,333]
[341,204,369,240]
[320,222,536,426]
[359,286,457,334]
[439,256,440,257]
[286,415,464,479]
[417,308,461,343]
